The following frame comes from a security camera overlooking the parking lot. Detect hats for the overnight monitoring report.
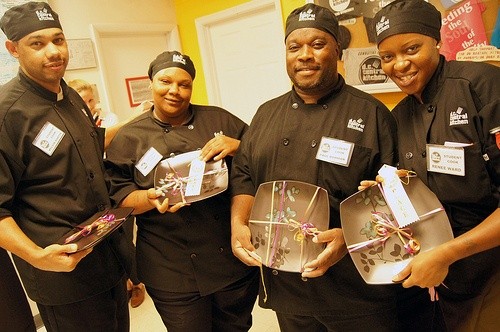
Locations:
[284,3,340,45]
[0,1,63,42]
[148,50,196,82]
[373,0,442,47]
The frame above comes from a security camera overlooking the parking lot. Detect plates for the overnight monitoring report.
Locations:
[153,150,227,205]
[244,180,330,273]
[54,209,133,251]
[340,173,453,285]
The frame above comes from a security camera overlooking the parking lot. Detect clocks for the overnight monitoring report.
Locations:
[65,38,97,71]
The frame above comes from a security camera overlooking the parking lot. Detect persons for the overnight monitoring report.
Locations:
[75,81,145,308]
[0,1,154,332]
[230,1,395,332]
[358,1,500,332]
[104,50,258,332]
